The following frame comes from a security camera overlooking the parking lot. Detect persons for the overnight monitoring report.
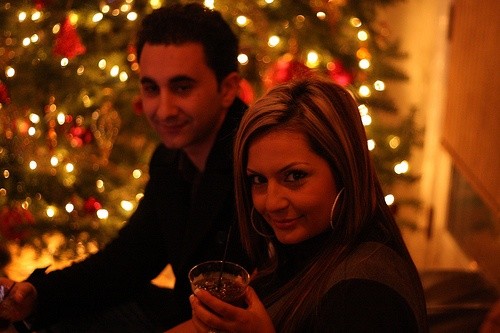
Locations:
[163,79,428,333]
[1,5,248,333]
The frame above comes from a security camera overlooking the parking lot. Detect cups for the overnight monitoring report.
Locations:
[189,260,251,307]
